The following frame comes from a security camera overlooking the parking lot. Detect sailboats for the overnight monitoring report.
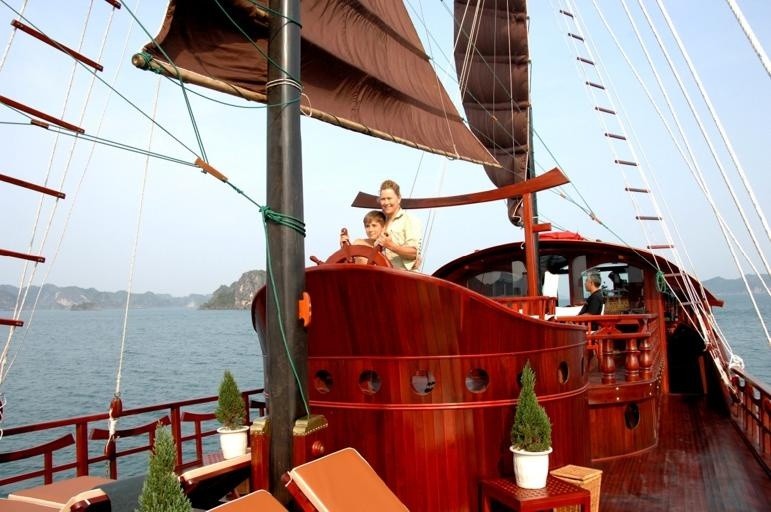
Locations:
[1,0,770,512]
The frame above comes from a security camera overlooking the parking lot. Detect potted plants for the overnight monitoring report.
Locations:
[218,370,251,460]
[508,357,556,493]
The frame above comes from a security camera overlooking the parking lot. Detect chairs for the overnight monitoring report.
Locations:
[213,446,415,509]
[0,453,252,512]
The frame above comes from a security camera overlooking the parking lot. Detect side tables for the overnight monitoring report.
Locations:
[477,474,591,511]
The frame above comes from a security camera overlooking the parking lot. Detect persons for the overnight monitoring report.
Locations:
[559,274,604,383]
[340,211,423,270]
[377,180,418,273]
[664,312,704,395]
